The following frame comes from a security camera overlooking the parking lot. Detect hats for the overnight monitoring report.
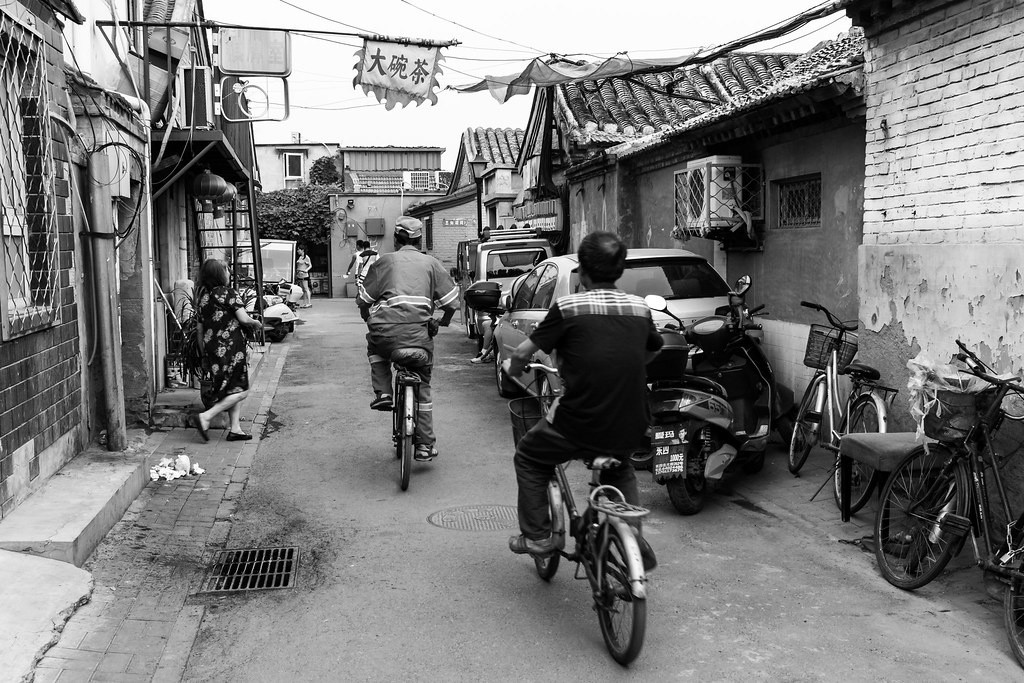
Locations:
[395,216,423,238]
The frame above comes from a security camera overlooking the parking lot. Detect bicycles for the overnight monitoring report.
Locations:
[501,364,650,665]
[377,347,432,490]
[788,300,899,515]
[874,340,1024,668]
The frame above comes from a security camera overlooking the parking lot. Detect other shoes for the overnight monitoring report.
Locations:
[300,304,312,309]
[193,414,210,441]
[470,352,491,363]
[226,432,252,441]
[491,350,495,361]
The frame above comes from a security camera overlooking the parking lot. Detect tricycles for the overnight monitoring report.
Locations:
[450,238,553,353]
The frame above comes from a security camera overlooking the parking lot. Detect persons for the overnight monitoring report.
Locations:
[355,215,460,462]
[297,244,312,308]
[499,232,664,556]
[357,241,380,294]
[346,240,364,286]
[193,259,262,441]
[470,320,495,363]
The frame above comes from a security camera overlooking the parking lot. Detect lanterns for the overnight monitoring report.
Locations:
[212,181,238,210]
[186,169,227,204]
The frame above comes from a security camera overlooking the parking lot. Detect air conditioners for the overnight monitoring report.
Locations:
[686,154,742,228]
[180,66,215,130]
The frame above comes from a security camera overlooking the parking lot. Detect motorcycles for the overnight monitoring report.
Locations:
[230,238,297,285]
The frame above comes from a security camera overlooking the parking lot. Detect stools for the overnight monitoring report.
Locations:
[840,426,960,557]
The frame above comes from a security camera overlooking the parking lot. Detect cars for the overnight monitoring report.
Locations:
[492,250,734,420]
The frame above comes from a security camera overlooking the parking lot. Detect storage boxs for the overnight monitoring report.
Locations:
[646,327,690,383]
[463,282,502,310]
[276,283,304,304]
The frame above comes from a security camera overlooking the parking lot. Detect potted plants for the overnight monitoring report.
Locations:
[167,280,264,385]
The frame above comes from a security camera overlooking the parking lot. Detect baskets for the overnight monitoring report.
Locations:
[924,390,977,441]
[507,395,559,447]
[804,324,858,375]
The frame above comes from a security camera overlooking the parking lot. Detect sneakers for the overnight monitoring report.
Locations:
[415,444,437,461]
[370,393,392,409]
[630,524,658,567]
[509,534,555,557]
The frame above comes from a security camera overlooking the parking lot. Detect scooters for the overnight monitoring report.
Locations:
[464,281,505,362]
[239,272,305,343]
[629,274,806,515]
